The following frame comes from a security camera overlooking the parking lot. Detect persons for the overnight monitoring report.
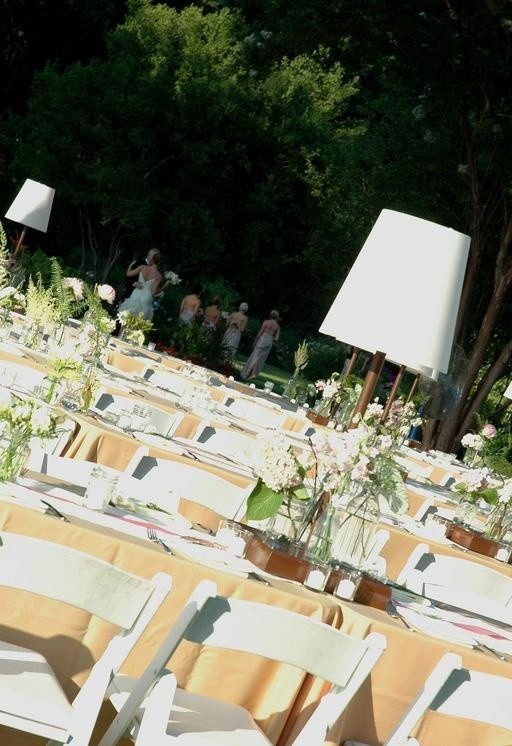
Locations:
[240,310,280,379]
[218,302,248,366]
[202,299,221,331]
[117,248,163,344]
[173,285,201,349]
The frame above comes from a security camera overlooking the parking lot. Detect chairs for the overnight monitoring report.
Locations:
[0,308,512,746]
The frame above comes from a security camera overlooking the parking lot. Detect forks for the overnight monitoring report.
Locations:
[146,526,174,556]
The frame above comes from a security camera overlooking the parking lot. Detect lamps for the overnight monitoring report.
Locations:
[244,209,472,610]
[5,179,56,271]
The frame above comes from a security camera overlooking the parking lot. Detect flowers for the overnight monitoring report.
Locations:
[0,220,115,349]
[159,270,181,290]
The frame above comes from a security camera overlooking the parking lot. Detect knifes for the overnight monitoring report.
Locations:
[472,637,506,661]
[36,496,67,520]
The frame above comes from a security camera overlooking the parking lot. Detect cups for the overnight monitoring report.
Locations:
[78,464,119,513]
[332,568,364,603]
[144,338,462,461]
[116,403,153,431]
[429,513,453,540]
[213,519,257,559]
[0,367,18,386]
[301,560,334,592]
